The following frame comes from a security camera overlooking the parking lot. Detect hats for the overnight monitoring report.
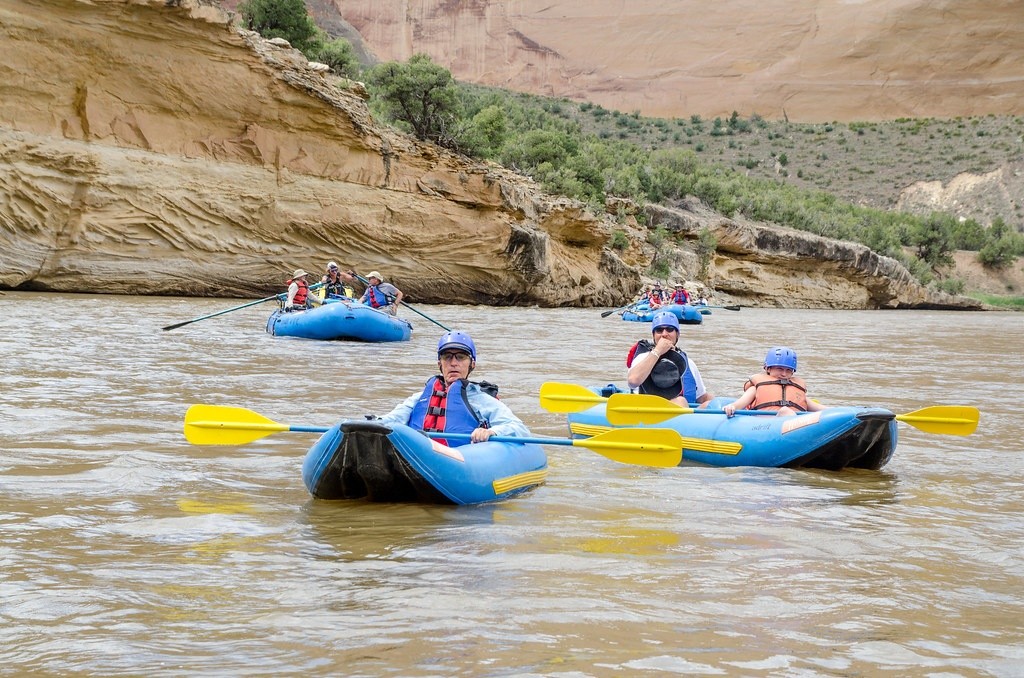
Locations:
[654,282,660,286]
[675,284,683,287]
[292,269,308,279]
[365,271,383,282]
[653,291,659,295]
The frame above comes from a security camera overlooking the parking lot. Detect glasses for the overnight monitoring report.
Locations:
[654,327,675,333]
[330,269,337,272]
[440,351,469,362]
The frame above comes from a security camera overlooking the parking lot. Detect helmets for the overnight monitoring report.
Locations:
[652,311,680,333]
[438,331,477,361]
[326,262,339,272]
[764,347,797,372]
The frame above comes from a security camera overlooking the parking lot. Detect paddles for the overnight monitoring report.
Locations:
[160,279,330,331]
[691,304,741,311]
[605,391,981,440]
[539,381,700,418]
[346,270,451,330]
[183,402,683,468]
[601,301,637,318]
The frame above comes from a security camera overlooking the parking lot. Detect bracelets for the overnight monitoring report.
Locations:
[395,302,398,307]
[650,349,659,359]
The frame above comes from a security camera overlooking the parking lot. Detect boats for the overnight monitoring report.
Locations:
[302,421,549,505]
[622,298,702,325]
[569,385,900,465]
[265,301,411,342]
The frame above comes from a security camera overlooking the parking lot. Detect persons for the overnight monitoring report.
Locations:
[641,282,708,310]
[370,332,531,447]
[357,270,404,317]
[284,269,324,312]
[320,262,359,301]
[722,347,828,416]
[626,312,715,410]
